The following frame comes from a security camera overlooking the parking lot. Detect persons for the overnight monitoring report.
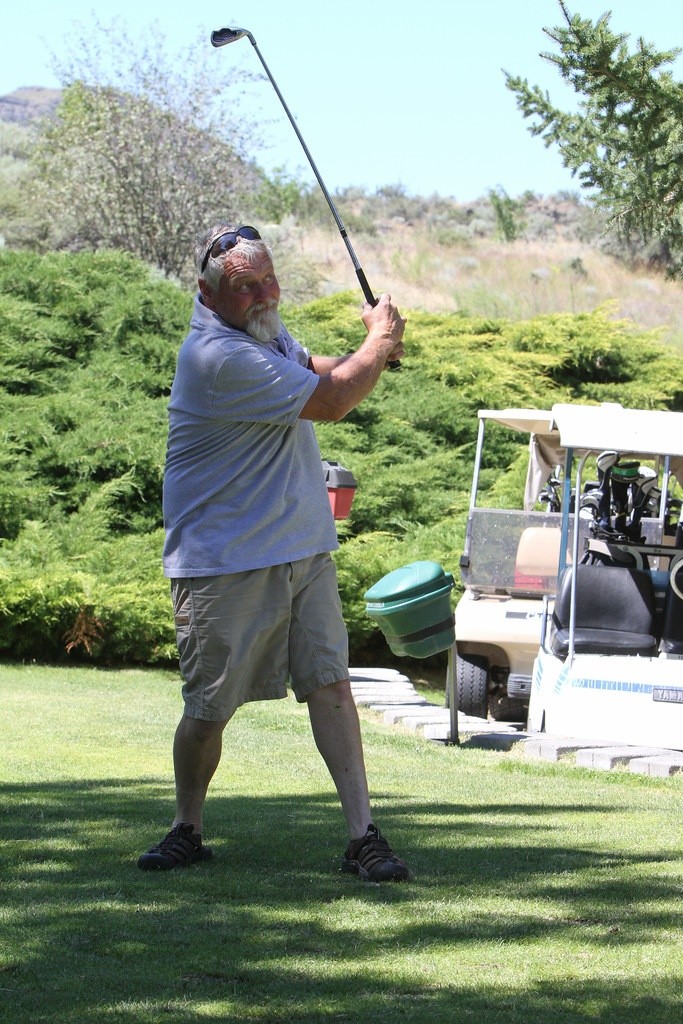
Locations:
[137,225,410,881]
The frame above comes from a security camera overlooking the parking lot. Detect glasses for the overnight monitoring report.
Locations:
[202,226,262,274]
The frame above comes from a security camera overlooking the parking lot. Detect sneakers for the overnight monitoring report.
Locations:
[138,822,212,871]
[342,823,409,882]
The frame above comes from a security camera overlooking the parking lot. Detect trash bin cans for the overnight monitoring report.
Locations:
[364,558,454,660]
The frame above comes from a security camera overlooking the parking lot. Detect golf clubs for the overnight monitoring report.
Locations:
[207,27,403,380]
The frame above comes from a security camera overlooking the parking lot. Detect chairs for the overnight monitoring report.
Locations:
[550,563,683,665]
